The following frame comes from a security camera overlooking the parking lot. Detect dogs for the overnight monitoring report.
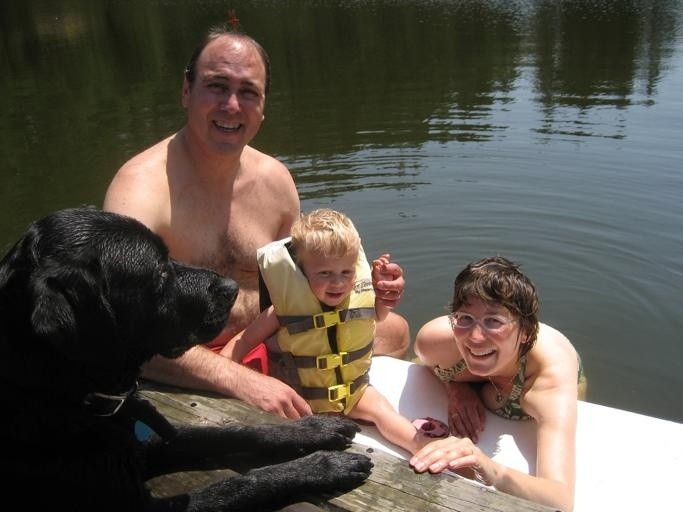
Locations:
[0,203,376,512]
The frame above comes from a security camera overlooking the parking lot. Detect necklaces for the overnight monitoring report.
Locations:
[488,373,517,405]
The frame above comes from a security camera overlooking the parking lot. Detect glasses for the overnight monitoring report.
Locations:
[448,312,517,332]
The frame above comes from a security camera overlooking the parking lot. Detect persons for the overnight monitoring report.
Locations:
[217,208,433,457]
[104,24,412,421]
[407,254,584,511]
